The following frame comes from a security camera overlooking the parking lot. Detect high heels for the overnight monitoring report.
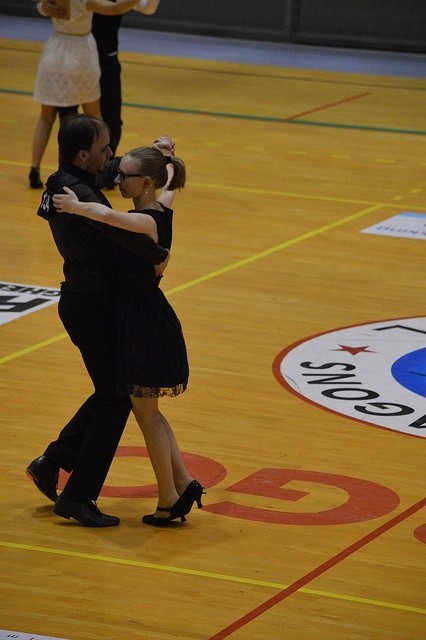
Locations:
[142,497,190,526]
[180,480,203,510]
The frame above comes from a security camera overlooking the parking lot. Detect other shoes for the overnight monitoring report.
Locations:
[29,166,44,188]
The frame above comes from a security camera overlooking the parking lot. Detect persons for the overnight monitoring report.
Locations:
[51,135,204,530]
[29,0,141,189]
[24,113,176,528]
[56,0,161,191]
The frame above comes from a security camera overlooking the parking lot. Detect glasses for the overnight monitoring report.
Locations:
[116,168,154,182]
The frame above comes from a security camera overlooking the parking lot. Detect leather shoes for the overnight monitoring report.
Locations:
[53,492,120,527]
[28,455,59,503]
